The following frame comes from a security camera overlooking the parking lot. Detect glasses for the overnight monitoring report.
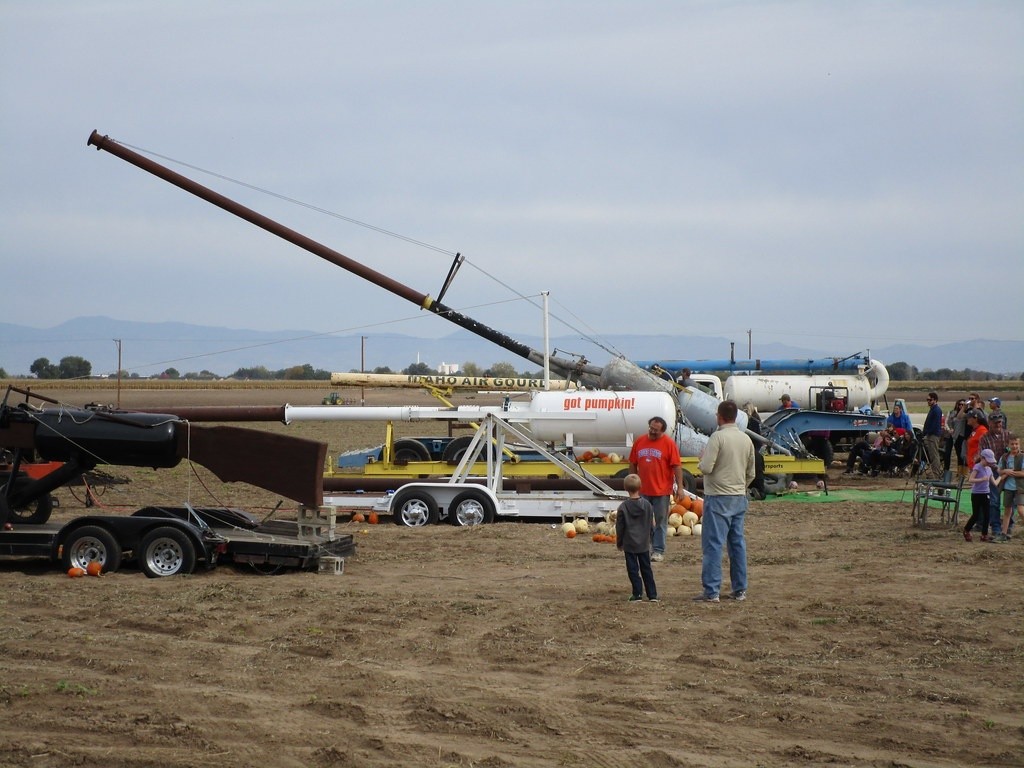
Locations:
[969,397,976,400]
[927,399,932,401]
[959,403,963,406]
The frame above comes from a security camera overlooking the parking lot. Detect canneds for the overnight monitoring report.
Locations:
[946,488,951,498]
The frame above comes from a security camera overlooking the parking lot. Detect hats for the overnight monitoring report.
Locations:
[989,412,1003,422]
[988,397,1001,408]
[779,394,790,401]
[969,408,986,419]
[981,449,997,463]
[896,428,905,436]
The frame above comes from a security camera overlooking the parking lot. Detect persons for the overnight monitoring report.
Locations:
[888,405,912,431]
[628,417,684,562]
[743,401,761,421]
[778,394,800,411]
[616,474,658,602]
[948,393,1007,471]
[843,423,918,478]
[692,401,755,602]
[978,414,1014,539]
[992,433,1024,543]
[922,393,942,479]
[949,449,1001,543]
[681,368,697,388]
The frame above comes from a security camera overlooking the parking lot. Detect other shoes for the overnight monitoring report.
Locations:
[843,468,853,475]
[979,534,993,542]
[990,532,1001,539]
[650,551,664,562]
[751,487,761,500]
[1006,528,1012,539]
[974,525,982,532]
[759,491,766,500]
[628,594,642,602]
[992,533,1008,543]
[692,593,719,603]
[650,597,662,603]
[727,590,746,600]
[963,527,973,541]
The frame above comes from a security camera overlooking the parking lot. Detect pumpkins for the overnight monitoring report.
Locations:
[667,494,704,536]
[68,562,102,577]
[561,510,618,543]
[576,448,628,463]
[353,511,378,524]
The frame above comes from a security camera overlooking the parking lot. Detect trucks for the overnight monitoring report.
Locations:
[671,347,891,460]
